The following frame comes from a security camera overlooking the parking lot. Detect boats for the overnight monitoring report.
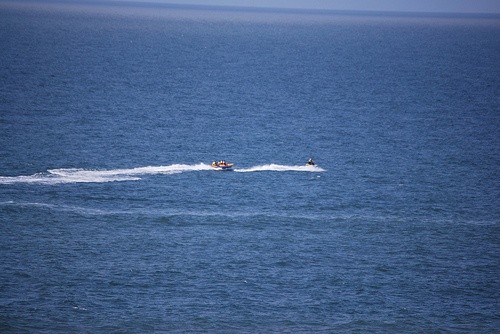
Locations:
[213,161,233,170]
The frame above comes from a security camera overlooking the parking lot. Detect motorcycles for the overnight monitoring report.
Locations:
[306,163,317,168]
